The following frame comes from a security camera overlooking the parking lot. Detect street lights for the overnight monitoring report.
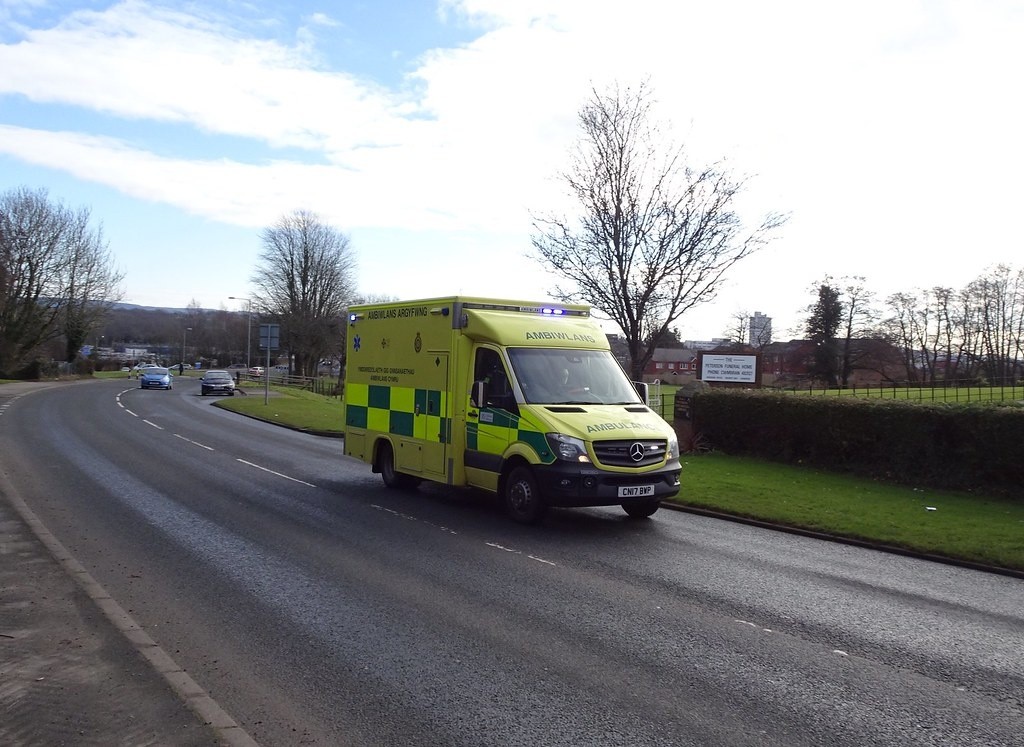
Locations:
[229,296,251,382]
[183,328,192,373]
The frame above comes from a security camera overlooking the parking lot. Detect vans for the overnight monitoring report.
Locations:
[343,296,682,529]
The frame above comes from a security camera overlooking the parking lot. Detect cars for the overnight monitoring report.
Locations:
[201,370,235,396]
[121,367,130,372]
[249,367,264,375]
[139,364,158,371]
[141,368,173,390]
[173,363,191,370]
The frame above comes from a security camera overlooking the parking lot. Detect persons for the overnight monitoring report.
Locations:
[553,367,577,395]
[236,370,241,384]
[178,360,184,375]
